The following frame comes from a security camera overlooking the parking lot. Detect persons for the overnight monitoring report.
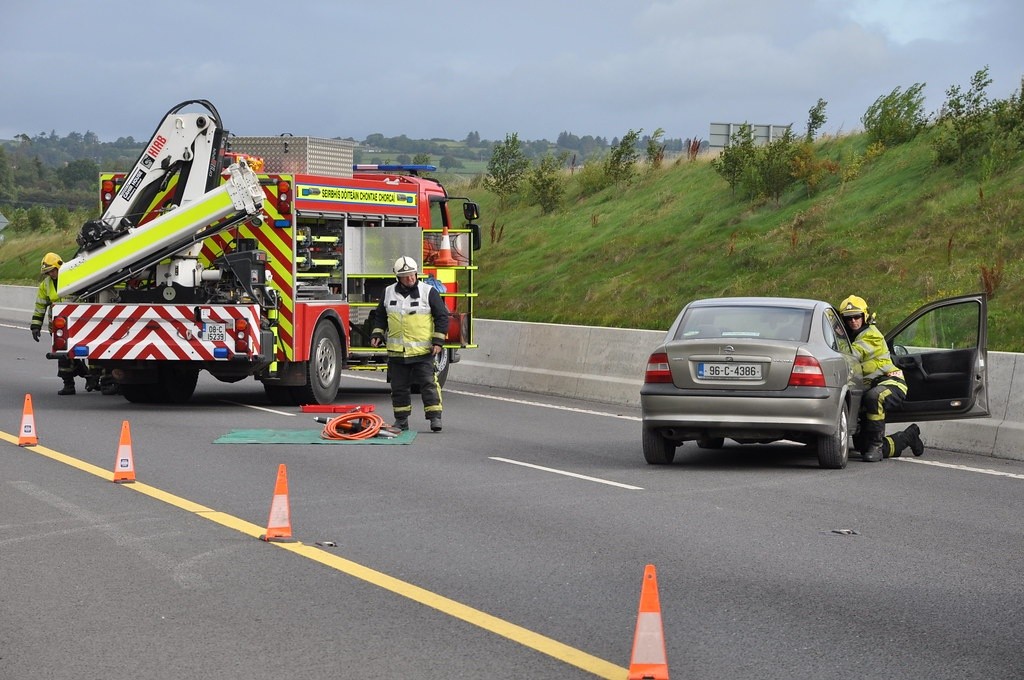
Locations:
[826,310,851,352]
[839,295,924,462]
[370,257,449,430]
[31,252,96,395]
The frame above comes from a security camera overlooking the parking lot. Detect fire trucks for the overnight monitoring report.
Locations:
[45,99,483,408]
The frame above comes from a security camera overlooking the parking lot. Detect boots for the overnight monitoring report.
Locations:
[895,423,924,457]
[85,373,100,392]
[862,433,885,462]
[57,382,76,396]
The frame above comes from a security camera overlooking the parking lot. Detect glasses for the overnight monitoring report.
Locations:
[844,314,863,321]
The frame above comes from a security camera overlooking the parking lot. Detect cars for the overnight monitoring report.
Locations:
[640,291,992,470]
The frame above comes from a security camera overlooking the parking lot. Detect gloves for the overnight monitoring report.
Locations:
[32,329,41,342]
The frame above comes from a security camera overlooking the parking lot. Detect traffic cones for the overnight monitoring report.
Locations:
[113,420,137,484]
[259,464,299,543]
[627,564,671,680]
[18,393,39,447]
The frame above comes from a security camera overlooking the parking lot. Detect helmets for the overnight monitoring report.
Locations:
[393,256,418,289]
[839,295,876,326]
[40,252,63,276]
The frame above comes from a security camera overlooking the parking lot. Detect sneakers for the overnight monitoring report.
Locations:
[392,418,409,431]
[430,417,443,433]
